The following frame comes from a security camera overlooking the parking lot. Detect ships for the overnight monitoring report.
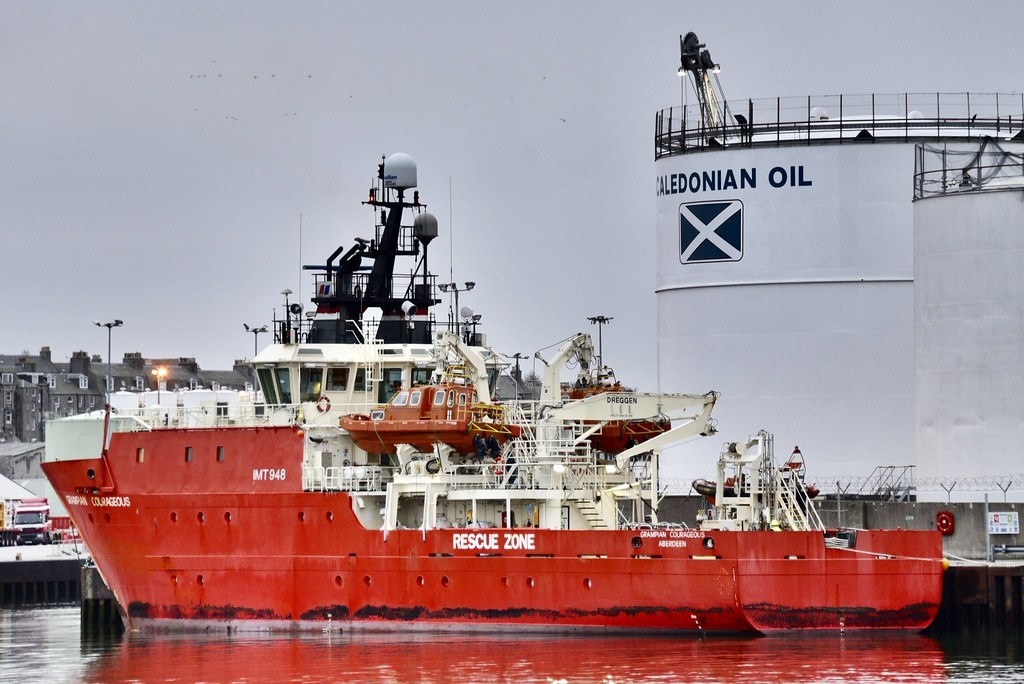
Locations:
[44,151,942,639]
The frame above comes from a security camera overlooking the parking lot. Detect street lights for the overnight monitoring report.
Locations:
[95,319,124,413]
[150,368,165,403]
[244,322,267,403]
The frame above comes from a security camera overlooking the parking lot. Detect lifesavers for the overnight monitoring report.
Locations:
[492,457,505,475]
[316,396,331,412]
[936,514,953,535]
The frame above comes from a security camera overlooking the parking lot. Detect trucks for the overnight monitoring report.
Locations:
[12,497,81,544]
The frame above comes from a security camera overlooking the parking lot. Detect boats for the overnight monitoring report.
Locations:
[340,383,524,457]
